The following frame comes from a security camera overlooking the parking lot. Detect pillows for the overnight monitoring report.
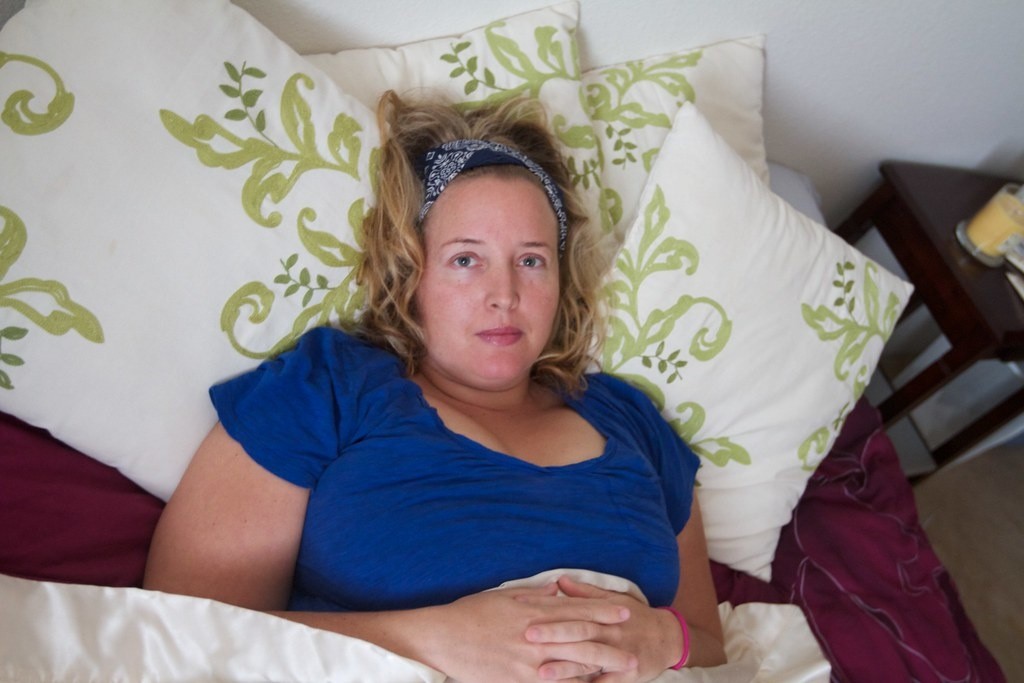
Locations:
[580,34,769,244]
[0,0,391,502]
[302,0,620,298]
[578,101,914,583]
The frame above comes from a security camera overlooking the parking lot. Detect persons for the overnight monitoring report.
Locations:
[143,90,727,683]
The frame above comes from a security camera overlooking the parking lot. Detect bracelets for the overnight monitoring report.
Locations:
[656,607,691,670]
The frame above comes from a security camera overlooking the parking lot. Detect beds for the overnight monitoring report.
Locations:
[0,161,1008,683]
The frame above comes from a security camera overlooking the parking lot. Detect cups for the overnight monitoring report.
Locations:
[956,182,1024,268]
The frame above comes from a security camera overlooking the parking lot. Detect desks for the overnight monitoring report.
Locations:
[831,160,1024,486]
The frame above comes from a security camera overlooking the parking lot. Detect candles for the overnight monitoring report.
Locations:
[956,183,1024,267]
